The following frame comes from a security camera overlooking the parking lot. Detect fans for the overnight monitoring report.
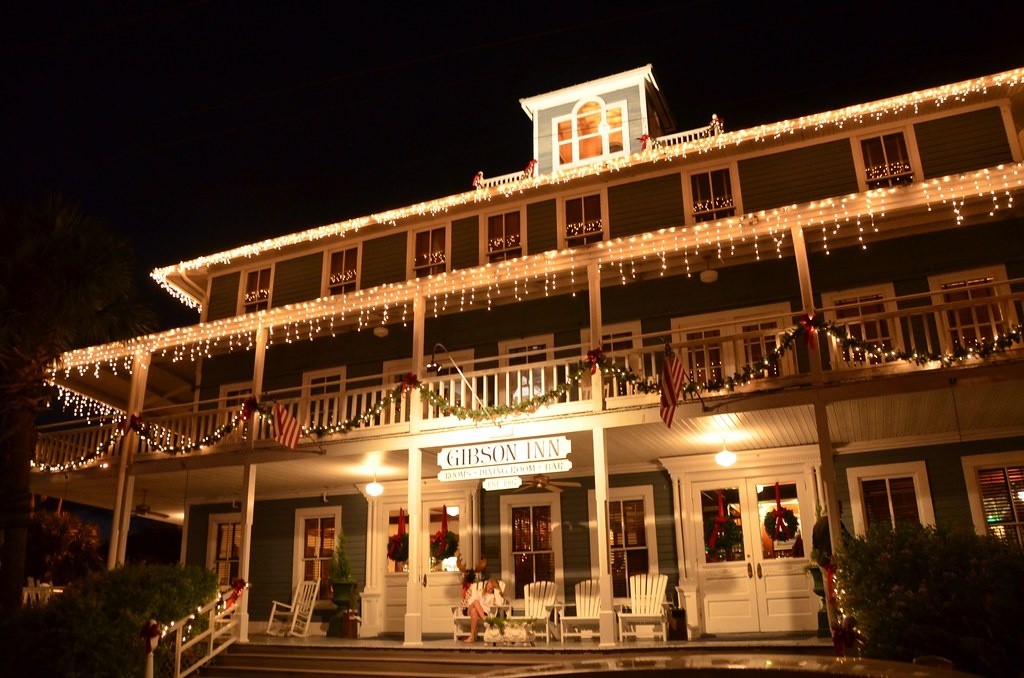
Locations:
[130,488,170,520]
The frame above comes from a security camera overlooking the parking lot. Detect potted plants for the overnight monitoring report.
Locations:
[803,503,835,639]
[326,528,362,639]
[482,616,538,647]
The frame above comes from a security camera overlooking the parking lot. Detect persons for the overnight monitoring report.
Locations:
[463,577,505,643]
[811,497,855,642]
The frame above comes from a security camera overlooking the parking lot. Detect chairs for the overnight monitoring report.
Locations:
[519,580,559,644]
[450,580,511,643]
[559,579,601,644]
[264,577,322,639]
[616,572,669,643]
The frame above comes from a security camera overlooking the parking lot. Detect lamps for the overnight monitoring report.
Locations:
[365,473,384,497]
[698,257,718,284]
[372,313,390,338]
[715,439,737,467]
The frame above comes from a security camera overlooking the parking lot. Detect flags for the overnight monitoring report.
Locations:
[268,401,303,452]
[658,341,685,429]
